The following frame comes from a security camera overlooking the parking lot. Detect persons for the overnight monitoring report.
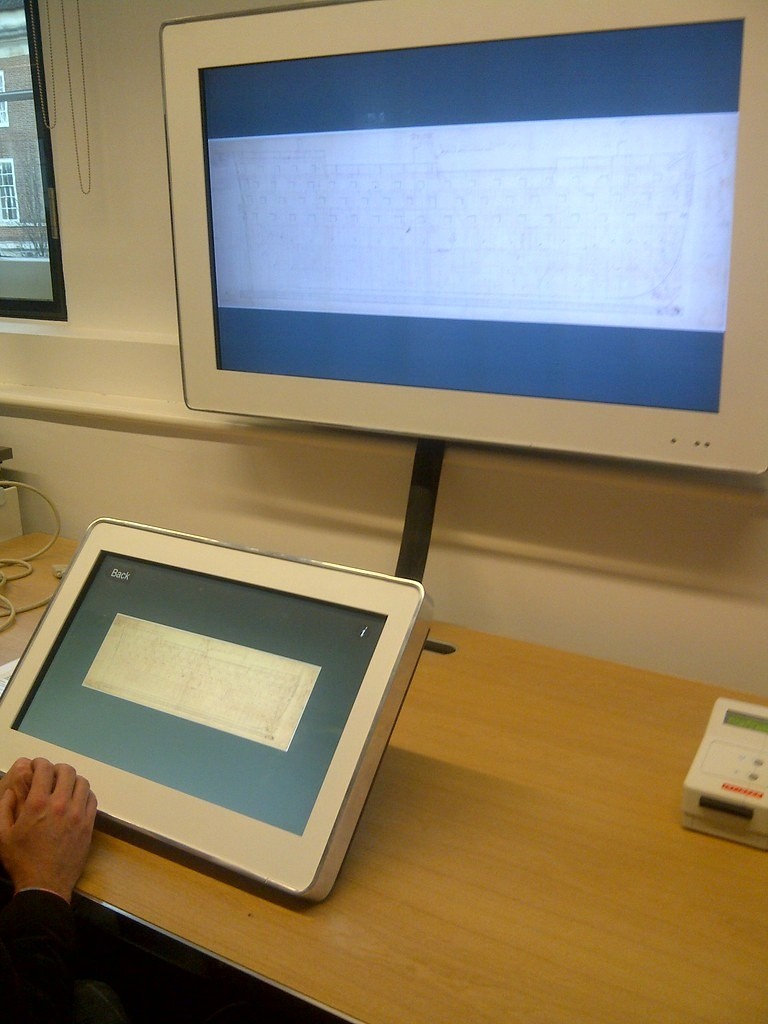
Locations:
[0,756,126,1024]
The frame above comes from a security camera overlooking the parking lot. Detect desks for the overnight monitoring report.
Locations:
[0,531,768,1024]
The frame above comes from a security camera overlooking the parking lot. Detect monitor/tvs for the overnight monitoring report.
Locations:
[159,0,768,475]
[0,517,435,902]
